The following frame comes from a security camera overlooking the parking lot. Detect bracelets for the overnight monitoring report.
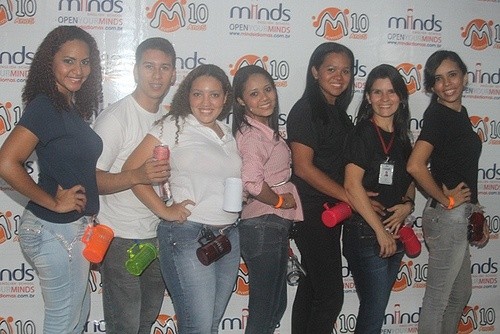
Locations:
[274,195,284,208]
[442,195,455,210]
[404,197,415,215]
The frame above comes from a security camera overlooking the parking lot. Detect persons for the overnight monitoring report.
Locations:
[233,65,304,334]
[122,64,243,334]
[90,37,176,334]
[344,63,416,334]
[0,25,103,334]
[286,42,386,334]
[405,49,489,334]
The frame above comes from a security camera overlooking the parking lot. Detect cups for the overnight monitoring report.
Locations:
[322,202,352,228]
[397,226,421,259]
[125,241,157,276]
[196,232,232,266]
[287,255,305,286]
[468,213,484,241]
[223,177,243,213]
[82,225,114,263]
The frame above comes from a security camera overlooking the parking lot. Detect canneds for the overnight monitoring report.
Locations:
[152,144,169,172]
